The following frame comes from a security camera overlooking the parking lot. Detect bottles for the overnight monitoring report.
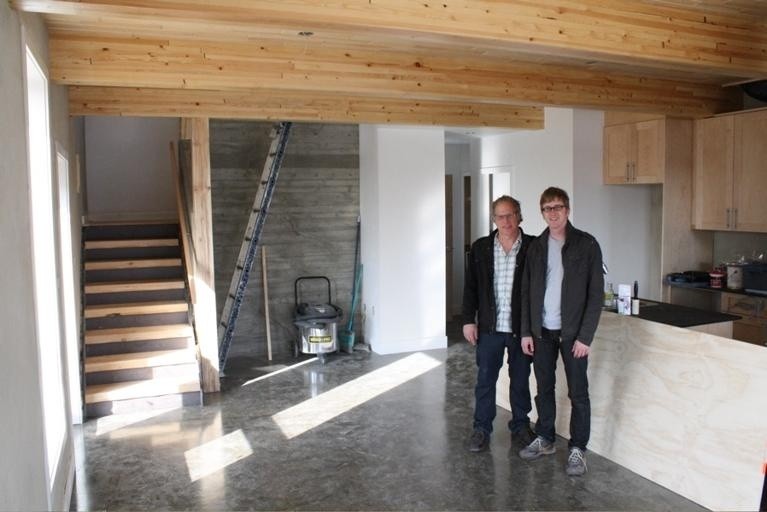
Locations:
[604,281,615,307]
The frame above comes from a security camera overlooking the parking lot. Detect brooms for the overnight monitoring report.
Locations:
[340,217,362,352]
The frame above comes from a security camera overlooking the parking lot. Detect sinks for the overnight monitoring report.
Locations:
[614,295,658,307]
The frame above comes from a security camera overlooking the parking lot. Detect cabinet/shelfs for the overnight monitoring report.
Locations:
[721,293,767,347]
[601,120,692,184]
[667,285,719,312]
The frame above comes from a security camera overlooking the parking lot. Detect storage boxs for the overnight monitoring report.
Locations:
[690,109,766,232]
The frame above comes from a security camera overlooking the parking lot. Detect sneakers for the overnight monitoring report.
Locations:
[468,427,588,476]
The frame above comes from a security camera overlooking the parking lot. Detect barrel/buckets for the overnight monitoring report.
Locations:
[726,262,749,290]
[709,272,725,288]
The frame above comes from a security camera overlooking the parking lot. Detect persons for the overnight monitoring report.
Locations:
[460,195,543,456]
[519,185,606,476]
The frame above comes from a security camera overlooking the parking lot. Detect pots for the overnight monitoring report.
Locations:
[721,260,764,291]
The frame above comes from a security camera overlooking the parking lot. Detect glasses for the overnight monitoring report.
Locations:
[541,204,566,213]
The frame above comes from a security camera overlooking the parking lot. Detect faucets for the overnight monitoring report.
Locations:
[602,262,610,277]
